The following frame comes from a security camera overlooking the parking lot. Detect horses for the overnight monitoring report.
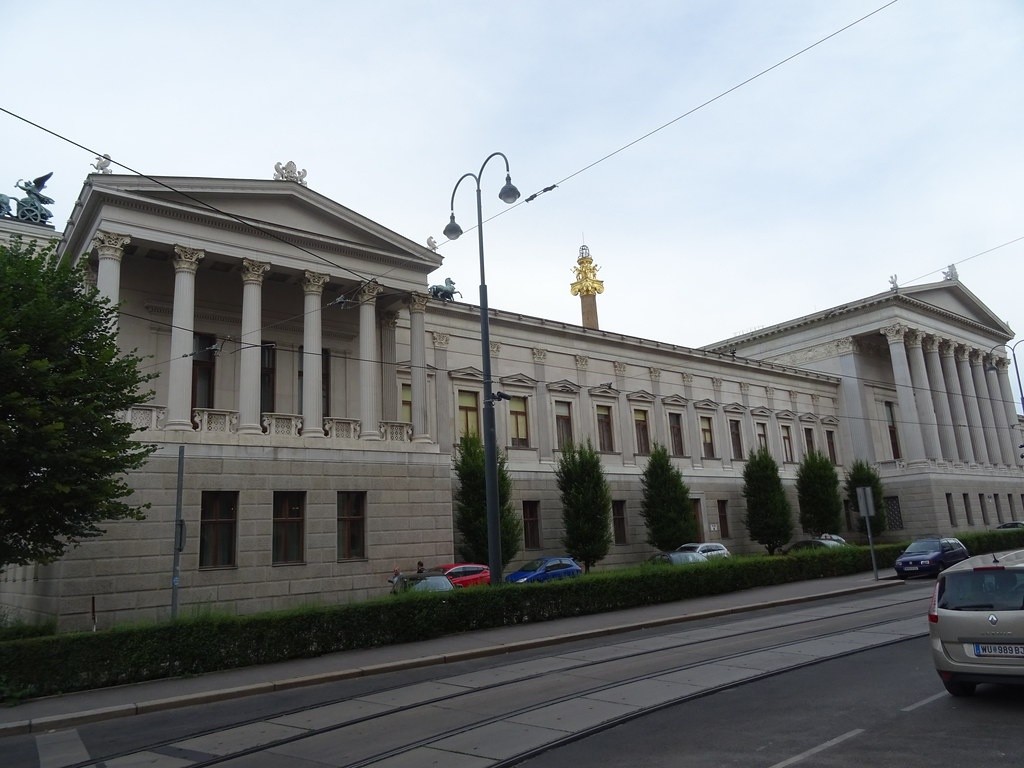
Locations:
[430,277,462,302]
[90,156,111,174]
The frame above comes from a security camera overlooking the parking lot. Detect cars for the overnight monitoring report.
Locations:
[643,550,709,566]
[783,538,842,555]
[927,548,1024,697]
[674,543,735,565]
[893,537,972,580]
[503,556,582,582]
[430,563,491,589]
[995,520,1024,528]
[389,570,456,599]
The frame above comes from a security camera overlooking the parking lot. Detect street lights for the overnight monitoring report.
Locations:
[442,152,520,588]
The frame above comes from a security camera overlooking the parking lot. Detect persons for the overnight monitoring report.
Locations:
[416,561,427,584]
[15,180,52,215]
[387,566,405,595]
